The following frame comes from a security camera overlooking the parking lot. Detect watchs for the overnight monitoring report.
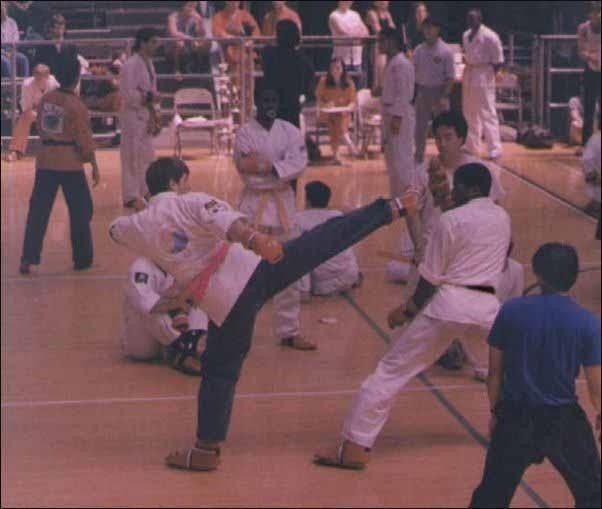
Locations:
[399,303,416,322]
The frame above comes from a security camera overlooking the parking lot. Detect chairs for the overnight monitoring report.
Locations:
[173,88,220,160]
[316,102,358,155]
[358,88,386,160]
[493,72,523,142]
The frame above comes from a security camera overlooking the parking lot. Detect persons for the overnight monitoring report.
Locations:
[438,241,525,370]
[329,0,369,89]
[35,15,80,82]
[96,65,121,128]
[367,0,396,87]
[120,28,164,210]
[378,27,417,199]
[6,63,60,161]
[312,163,512,469]
[577,9,602,146]
[407,2,428,48]
[116,255,209,377]
[410,17,457,164]
[256,17,316,192]
[263,1,303,50]
[107,156,421,471]
[111,47,128,66]
[232,83,316,351]
[582,130,601,202]
[163,0,212,82]
[21,54,100,273]
[461,10,505,159]
[569,96,599,144]
[404,111,505,305]
[6,1,33,26]
[297,179,363,303]
[213,1,259,123]
[1,6,30,77]
[468,242,602,509]
[316,57,358,165]
